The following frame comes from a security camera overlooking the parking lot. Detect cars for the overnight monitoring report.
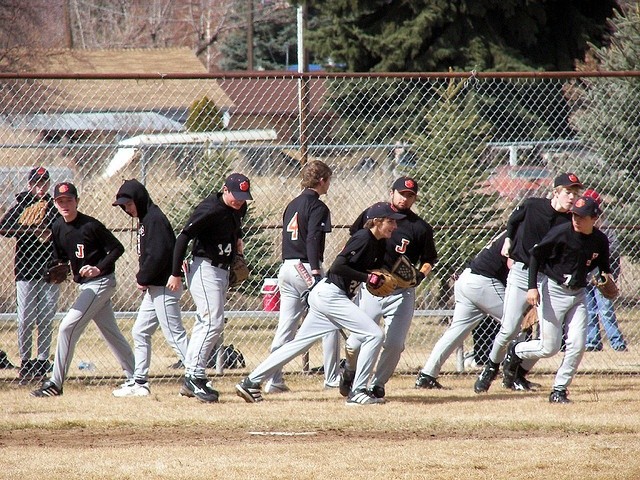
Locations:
[462,165,556,200]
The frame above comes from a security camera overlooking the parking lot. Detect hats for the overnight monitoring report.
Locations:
[583,190,601,205]
[225,173,253,200]
[392,177,417,194]
[112,197,132,206]
[28,167,50,182]
[367,202,407,220]
[54,183,77,200]
[555,173,582,188]
[569,197,598,217]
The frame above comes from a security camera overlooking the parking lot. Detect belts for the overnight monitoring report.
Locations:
[209,260,230,270]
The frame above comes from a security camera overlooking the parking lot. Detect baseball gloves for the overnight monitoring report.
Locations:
[392,256,418,288]
[43,259,69,283]
[595,273,619,299]
[230,256,248,285]
[367,268,396,296]
[520,307,539,328]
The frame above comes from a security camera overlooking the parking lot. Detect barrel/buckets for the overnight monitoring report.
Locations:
[260,278,280,311]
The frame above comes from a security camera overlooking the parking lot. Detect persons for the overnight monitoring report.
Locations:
[502,196,621,405]
[339,175,437,399]
[262,160,345,396]
[0,166,63,381]
[415,227,543,392]
[473,173,584,392]
[235,201,407,405]
[582,188,628,353]
[165,173,253,402]
[111,178,192,398]
[29,182,136,397]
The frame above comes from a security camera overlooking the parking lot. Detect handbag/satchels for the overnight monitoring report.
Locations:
[207,345,245,370]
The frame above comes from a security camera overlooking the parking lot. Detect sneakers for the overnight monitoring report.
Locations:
[587,347,602,351]
[503,339,523,381]
[20,364,32,377]
[235,378,263,403]
[502,365,531,390]
[185,378,218,401]
[324,372,344,388]
[178,381,213,397]
[474,358,500,394]
[616,345,627,351]
[503,381,541,388]
[345,388,385,406]
[30,379,63,397]
[268,378,290,392]
[112,382,150,396]
[34,364,45,377]
[340,366,356,396]
[415,372,443,389]
[180,379,219,398]
[368,386,385,398]
[549,385,570,403]
